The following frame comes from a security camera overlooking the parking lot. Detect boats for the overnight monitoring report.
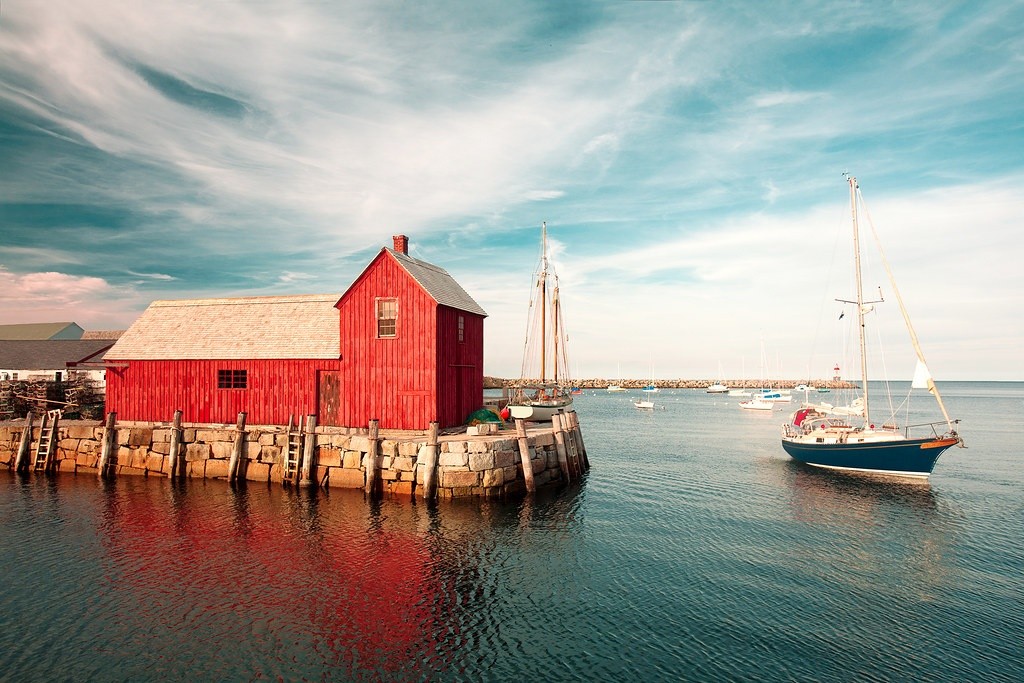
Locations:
[570,386,581,395]
[642,385,658,393]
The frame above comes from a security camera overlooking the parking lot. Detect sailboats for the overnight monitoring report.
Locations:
[782,172,960,476]
[706,338,831,410]
[506,221,573,423]
[607,362,626,392]
[634,351,655,408]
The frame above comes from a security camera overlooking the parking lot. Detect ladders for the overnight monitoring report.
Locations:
[32,413,59,473]
[283,414,303,485]
